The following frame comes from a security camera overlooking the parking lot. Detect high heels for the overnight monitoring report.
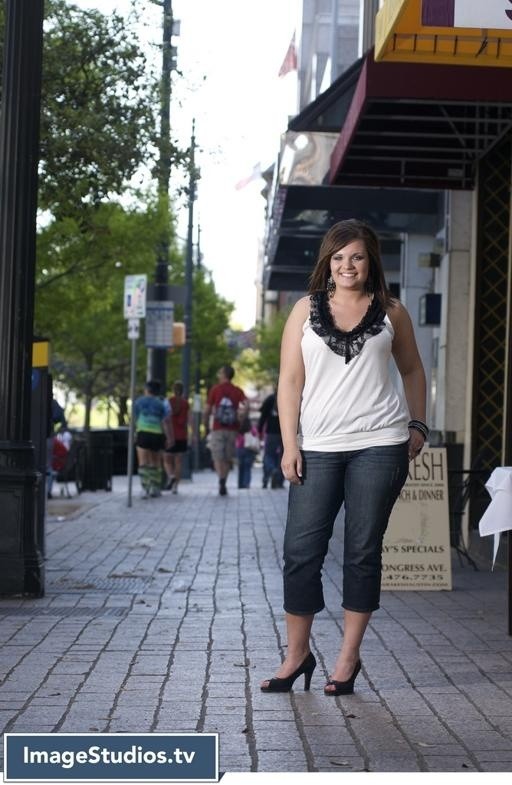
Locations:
[260,651,317,692]
[323,658,362,696]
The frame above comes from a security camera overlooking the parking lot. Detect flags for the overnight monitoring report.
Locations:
[279,34,297,76]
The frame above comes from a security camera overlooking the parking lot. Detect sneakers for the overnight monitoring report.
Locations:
[132,475,291,502]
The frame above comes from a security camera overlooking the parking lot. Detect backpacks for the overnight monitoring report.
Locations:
[215,385,238,426]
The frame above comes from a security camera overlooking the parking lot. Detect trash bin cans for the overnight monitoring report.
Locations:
[72,432,113,496]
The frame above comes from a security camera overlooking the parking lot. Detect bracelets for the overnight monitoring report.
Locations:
[407,421,430,440]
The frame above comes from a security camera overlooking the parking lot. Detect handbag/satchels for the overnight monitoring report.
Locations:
[243,430,261,454]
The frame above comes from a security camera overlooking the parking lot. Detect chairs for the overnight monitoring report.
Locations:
[46,442,82,498]
[447,468,479,570]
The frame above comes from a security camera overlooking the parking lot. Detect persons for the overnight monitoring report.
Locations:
[129,380,174,500]
[201,363,249,496]
[258,218,430,697]
[46,395,66,499]
[233,415,260,489]
[163,380,196,496]
[55,425,73,452]
[255,385,282,489]
